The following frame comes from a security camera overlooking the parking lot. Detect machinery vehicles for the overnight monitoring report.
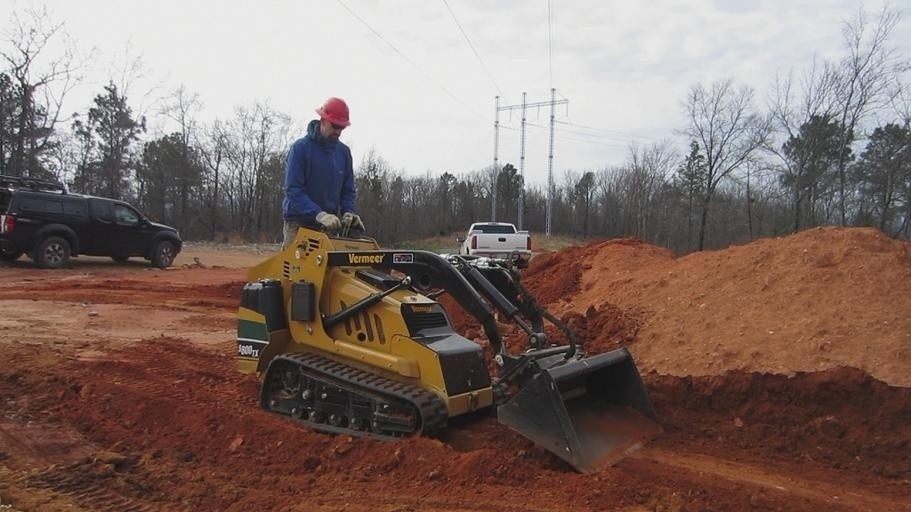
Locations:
[236,229,662,475]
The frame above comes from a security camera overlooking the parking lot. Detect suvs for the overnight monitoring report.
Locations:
[1,176,182,269]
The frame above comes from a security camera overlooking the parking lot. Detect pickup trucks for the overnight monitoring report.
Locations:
[458,222,533,269]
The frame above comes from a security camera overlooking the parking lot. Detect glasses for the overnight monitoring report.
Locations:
[330,123,345,131]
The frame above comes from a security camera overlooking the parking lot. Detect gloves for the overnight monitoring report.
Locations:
[315,211,342,230]
[342,212,366,232]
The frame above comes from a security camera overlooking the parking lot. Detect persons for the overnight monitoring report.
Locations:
[278,97,365,252]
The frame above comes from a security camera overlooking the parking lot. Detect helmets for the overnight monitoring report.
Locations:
[315,97,352,127]
